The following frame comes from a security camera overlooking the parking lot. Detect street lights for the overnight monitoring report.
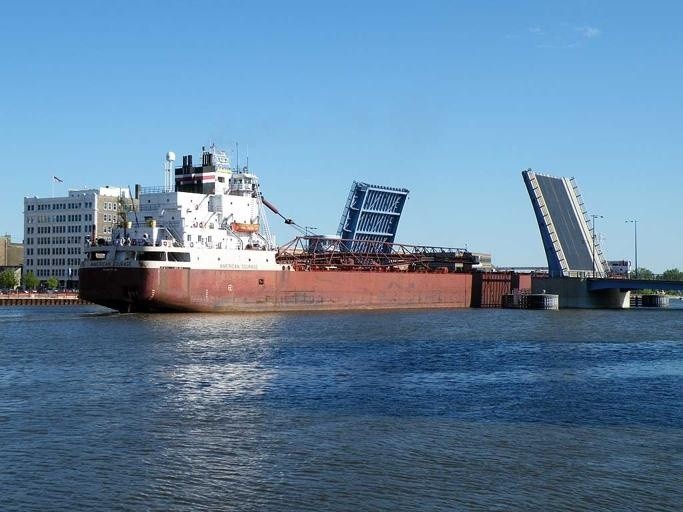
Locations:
[589,213,605,279]
[625,219,639,278]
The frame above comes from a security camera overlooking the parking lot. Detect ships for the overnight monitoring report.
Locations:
[77,138,478,313]
